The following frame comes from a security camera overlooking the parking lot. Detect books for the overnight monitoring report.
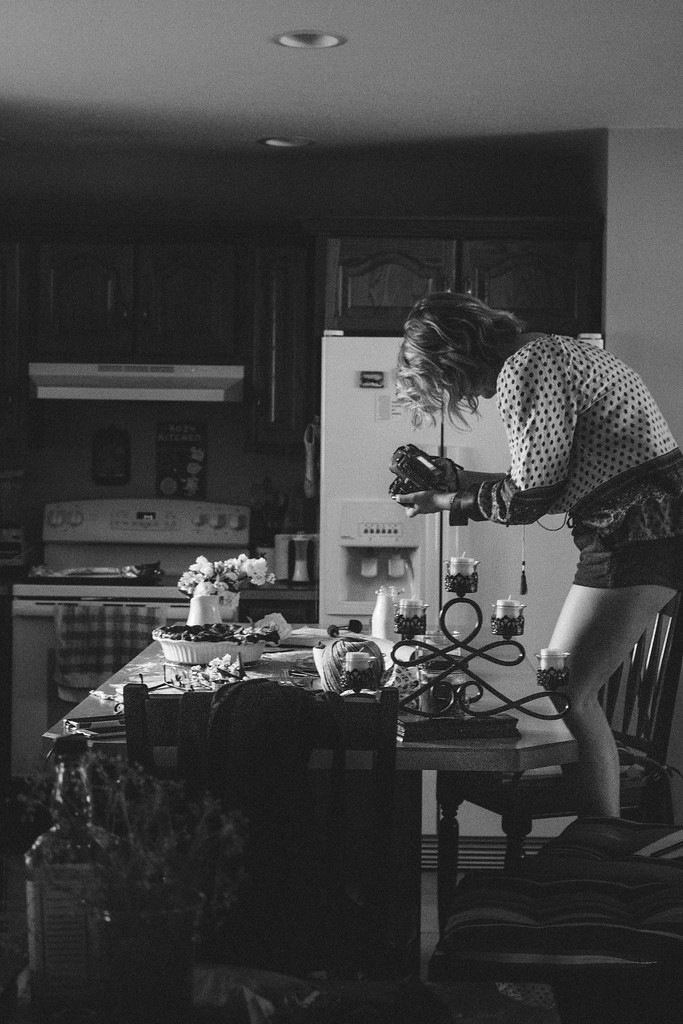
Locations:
[397,711,522,743]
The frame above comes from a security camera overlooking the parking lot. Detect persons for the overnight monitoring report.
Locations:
[392,291,683,818]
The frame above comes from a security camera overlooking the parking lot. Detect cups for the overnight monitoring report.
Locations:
[255,548,275,580]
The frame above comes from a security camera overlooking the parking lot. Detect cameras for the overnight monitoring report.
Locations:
[388,443,445,507]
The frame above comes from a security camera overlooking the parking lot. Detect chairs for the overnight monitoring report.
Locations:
[439,594,683,941]
[123,679,425,1023]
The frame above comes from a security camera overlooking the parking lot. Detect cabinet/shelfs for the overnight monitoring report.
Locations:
[0,219,604,459]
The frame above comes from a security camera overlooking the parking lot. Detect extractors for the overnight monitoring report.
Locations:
[27,359,247,403]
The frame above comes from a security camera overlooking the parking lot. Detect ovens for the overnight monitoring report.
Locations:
[9,618,171,785]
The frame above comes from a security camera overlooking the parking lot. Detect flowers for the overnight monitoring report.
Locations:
[178,553,273,595]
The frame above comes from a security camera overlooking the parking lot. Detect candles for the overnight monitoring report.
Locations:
[448,551,474,577]
[540,645,564,671]
[400,594,422,618]
[497,594,524,619]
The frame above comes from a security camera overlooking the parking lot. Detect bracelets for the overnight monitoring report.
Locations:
[450,493,458,506]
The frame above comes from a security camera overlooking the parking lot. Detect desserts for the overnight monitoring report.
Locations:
[151,624,281,665]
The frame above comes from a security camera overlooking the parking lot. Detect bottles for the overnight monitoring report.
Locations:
[371,586,405,640]
[23,742,129,999]
[416,629,469,725]
[185,596,222,626]
[288,532,313,590]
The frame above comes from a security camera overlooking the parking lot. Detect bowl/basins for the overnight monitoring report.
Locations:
[151,635,267,666]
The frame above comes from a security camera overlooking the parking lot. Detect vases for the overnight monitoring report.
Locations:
[186,595,221,625]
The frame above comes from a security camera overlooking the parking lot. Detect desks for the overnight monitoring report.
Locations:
[44,641,582,978]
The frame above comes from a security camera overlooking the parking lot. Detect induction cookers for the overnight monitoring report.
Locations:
[10,496,253,620]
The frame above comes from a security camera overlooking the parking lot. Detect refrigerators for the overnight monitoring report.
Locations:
[314,332,605,870]
